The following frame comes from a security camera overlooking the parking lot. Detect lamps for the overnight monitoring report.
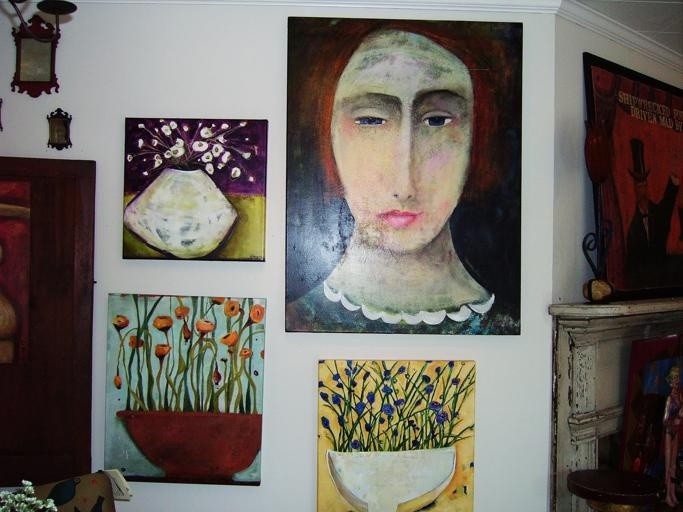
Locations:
[45,107,73,151]
[7,0,78,100]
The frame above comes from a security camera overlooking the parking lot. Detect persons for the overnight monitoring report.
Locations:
[654,362,681,510]
[285,18,520,334]
[621,131,680,292]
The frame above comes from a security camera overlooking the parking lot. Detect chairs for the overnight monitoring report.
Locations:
[27,469,114,511]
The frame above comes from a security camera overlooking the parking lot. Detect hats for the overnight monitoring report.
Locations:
[628,137,651,181]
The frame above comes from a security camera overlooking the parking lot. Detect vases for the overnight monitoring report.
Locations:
[324,445,457,512]
[114,409,261,482]
[122,168,241,260]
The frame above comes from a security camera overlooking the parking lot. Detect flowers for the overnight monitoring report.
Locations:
[124,119,260,181]
[0,477,59,512]
[317,362,478,453]
[109,294,266,412]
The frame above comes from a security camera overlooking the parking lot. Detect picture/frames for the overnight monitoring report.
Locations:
[581,49,683,303]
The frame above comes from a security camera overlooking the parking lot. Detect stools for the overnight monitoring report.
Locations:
[566,468,669,511]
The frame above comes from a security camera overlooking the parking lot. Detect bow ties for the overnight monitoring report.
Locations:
[637,211,655,224]
